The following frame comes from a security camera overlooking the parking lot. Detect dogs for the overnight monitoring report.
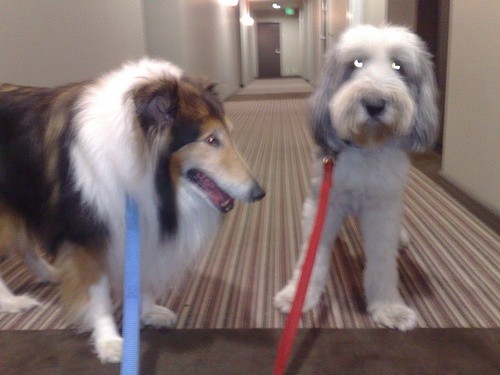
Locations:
[270,24,438,333]
[0,55,265,363]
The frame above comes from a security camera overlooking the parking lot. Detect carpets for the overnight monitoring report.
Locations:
[240,77,313,94]
[1,97,499,330]
